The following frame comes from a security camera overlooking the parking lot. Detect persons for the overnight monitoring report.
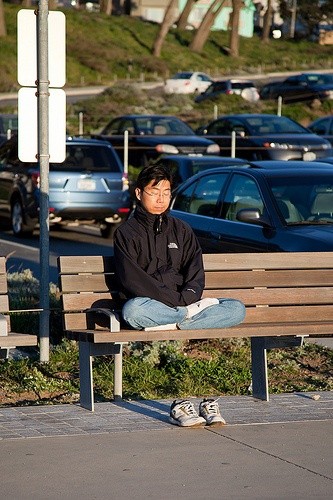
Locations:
[114,166,246,332]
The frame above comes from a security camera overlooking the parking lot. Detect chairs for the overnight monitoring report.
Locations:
[190,193,333,224]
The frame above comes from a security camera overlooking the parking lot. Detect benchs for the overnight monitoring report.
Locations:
[0,257,39,347]
[56,252,333,410]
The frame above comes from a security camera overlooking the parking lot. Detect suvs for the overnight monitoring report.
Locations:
[194,79,260,104]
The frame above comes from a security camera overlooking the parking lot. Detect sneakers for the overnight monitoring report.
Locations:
[199,398,226,426]
[168,399,207,427]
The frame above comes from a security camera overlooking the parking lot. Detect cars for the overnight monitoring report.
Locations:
[156,156,333,255]
[161,72,213,94]
[258,73,332,103]
[90,114,221,169]
[0,135,129,241]
[192,113,332,163]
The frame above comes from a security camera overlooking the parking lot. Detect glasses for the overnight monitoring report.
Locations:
[142,189,172,198]
